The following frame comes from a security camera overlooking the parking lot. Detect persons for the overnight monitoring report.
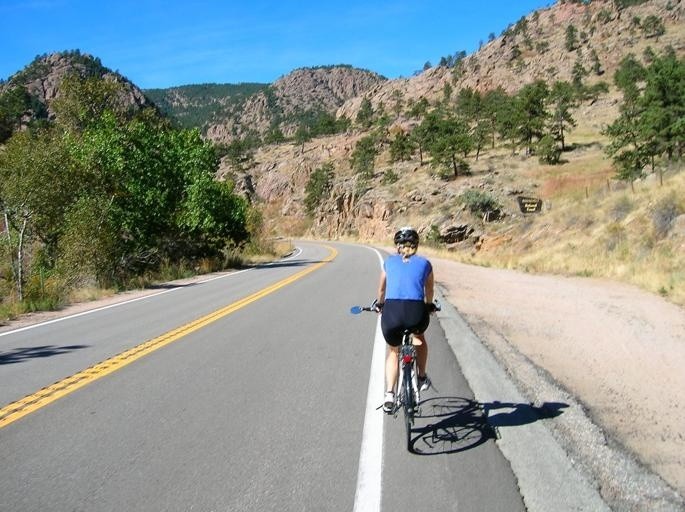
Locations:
[370,227,435,415]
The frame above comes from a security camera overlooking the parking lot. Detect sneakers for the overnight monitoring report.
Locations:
[418,377,431,391]
[382,391,395,412]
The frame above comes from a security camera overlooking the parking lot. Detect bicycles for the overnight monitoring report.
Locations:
[362,299,441,451]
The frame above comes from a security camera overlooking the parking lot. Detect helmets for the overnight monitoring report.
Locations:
[394,227,419,243]
